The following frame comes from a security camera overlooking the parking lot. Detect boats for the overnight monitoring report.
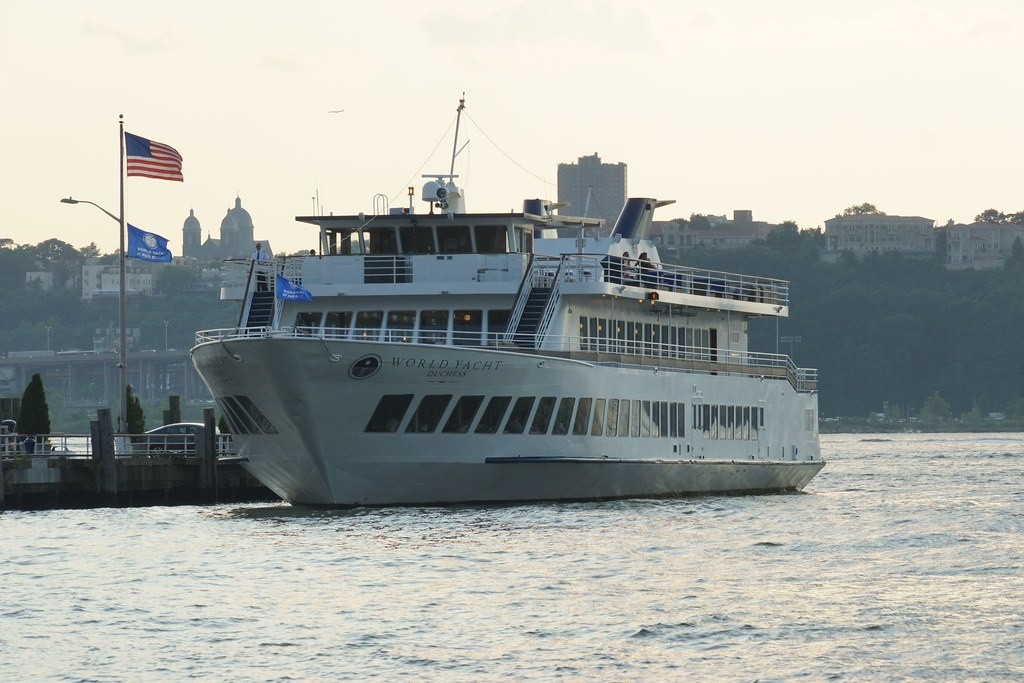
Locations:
[187,92,827,508]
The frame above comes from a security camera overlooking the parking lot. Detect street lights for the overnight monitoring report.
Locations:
[65,198,128,433]
[45,326,51,351]
[163,320,170,350]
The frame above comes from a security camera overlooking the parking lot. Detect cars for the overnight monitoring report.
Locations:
[141,422,223,456]
[824,418,839,422]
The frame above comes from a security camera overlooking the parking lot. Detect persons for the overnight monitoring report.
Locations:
[23,432,36,454]
[621,252,631,278]
[309,249,316,256]
[386,412,585,435]
[251,242,270,292]
[634,252,652,271]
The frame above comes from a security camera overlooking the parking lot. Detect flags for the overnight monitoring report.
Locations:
[276,274,314,302]
[124,131,184,182]
[126,222,173,263]
